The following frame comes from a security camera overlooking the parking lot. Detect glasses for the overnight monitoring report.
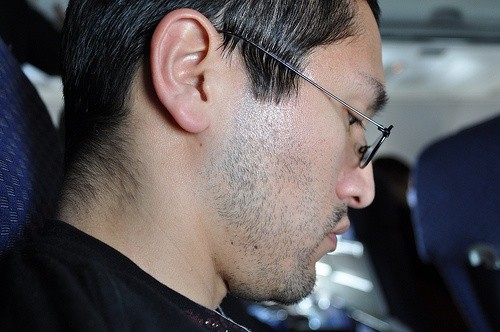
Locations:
[213,28,393,168]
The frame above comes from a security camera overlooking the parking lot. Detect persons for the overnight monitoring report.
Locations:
[0,0,390,332]
